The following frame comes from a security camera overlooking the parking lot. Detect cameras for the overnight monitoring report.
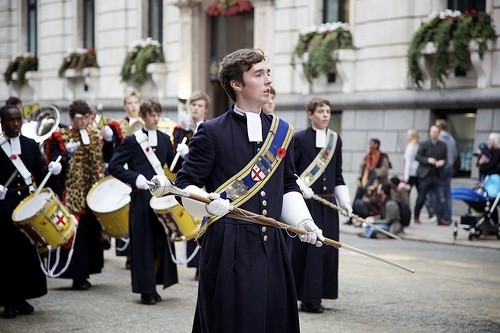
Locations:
[434,161,439,168]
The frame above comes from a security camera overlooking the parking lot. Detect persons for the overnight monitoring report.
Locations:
[108,98,189,306]
[0,84,500,318]
[175,48,326,333]
[293,98,353,313]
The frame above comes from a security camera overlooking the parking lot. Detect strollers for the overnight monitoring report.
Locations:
[448,172,499,241]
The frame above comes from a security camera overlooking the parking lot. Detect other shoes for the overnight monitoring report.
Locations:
[3,305,17,318]
[300,302,324,313]
[415,219,420,224]
[439,219,450,225]
[15,300,34,314]
[72,281,92,290]
[142,292,162,304]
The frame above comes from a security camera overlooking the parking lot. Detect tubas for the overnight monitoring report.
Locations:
[22,105,61,160]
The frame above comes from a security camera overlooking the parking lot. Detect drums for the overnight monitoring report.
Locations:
[86,175,133,237]
[149,195,203,242]
[12,186,77,253]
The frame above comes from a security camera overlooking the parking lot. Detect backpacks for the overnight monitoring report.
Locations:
[393,196,410,226]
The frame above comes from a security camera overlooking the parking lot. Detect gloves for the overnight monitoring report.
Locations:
[48,161,62,175]
[176,143,189,158]
[298,219,325,248]
[206,192,231,216]
[0,185,8,199]
[340,202,353,218]
[302,186,313,200]
[136,174,150,190]
[66,138,80,153]
[101,125,113,142]
